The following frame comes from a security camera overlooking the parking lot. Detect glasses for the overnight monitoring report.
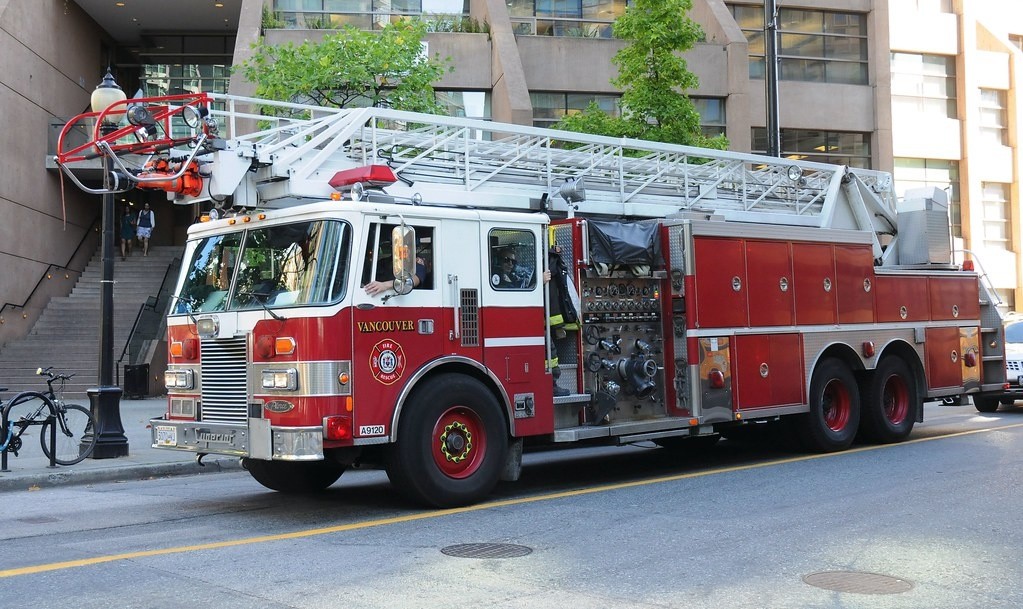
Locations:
[504,256,517,265]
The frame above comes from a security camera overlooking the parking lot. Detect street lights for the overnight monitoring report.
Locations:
[78,68,129,460]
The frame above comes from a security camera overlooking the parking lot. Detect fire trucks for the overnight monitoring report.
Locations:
[53,89,1009,511]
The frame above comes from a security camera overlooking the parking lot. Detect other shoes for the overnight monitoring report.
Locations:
[122,257,125,260]
[553,383,570,396]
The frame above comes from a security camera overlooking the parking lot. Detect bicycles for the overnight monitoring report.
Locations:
[1,365,100,467]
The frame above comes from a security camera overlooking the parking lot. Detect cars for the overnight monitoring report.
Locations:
[971,311,1023,413]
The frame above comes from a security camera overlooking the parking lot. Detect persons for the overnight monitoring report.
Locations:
[119,204,136,261]
[137,202,155,257]
[542,269,571,397]
[491,246,525,288]
[362,233,427,297]
